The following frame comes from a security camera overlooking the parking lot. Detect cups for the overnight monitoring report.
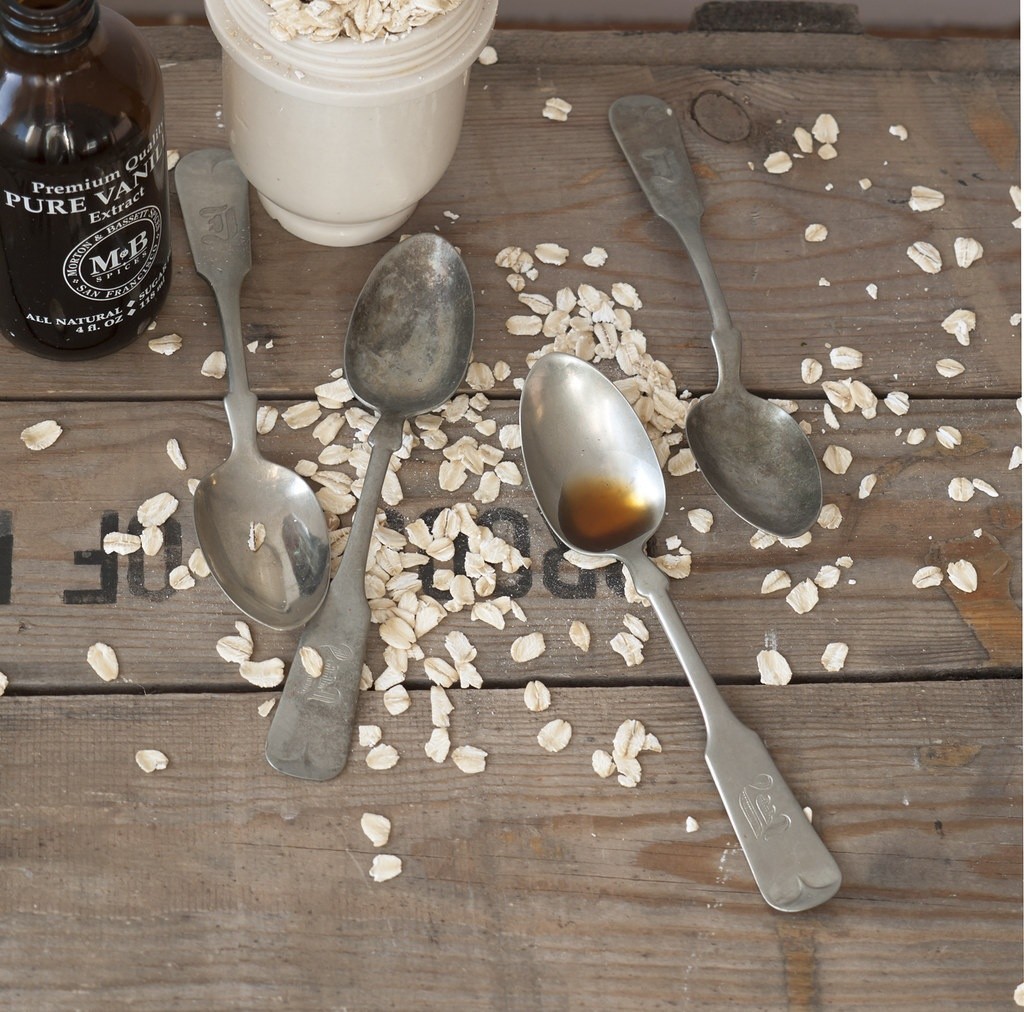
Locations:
[203,0,498,248]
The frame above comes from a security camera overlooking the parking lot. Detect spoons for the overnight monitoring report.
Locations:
[518,350,844,915]
[174,146,334,632]
[606,93,824,536]
[262,231,474,779]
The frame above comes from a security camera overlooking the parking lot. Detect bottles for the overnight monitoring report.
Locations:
[1,1,171,361]
